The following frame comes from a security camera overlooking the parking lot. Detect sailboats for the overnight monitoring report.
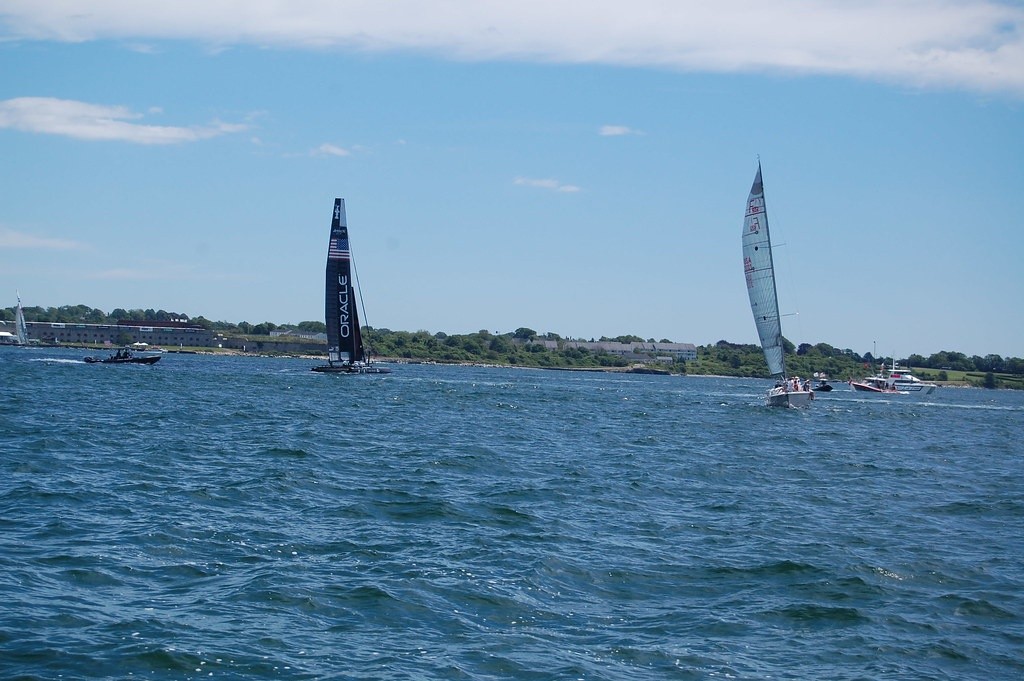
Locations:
[14,290,31,347]
[310,197,392,375]
[742,154,810,410]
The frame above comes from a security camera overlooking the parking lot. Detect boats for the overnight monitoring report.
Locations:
[846,341,938,395]
[167,349,197,354]
[812,378,834,391]
[84,355,162,366]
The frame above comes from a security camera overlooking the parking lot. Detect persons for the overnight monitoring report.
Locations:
[775,375,814,402]
[115,348,131,359]
